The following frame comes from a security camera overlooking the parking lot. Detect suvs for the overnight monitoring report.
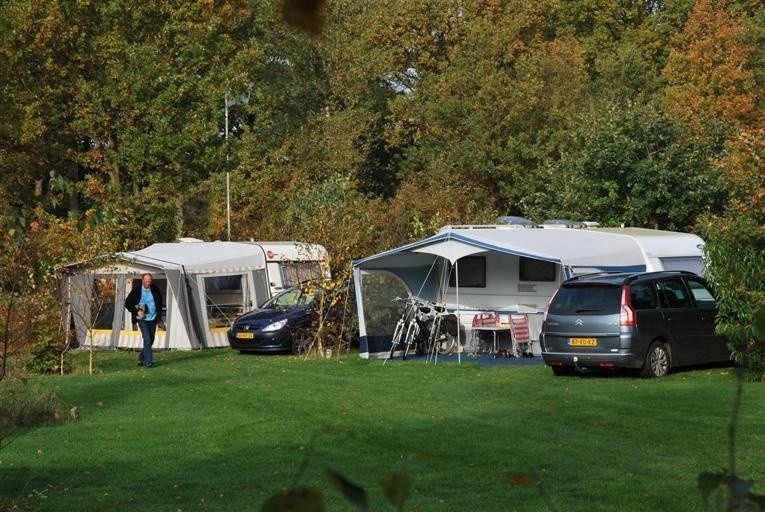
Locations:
[535,267,742,379]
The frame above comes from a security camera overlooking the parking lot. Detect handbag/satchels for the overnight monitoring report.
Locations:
[135,304,146,311]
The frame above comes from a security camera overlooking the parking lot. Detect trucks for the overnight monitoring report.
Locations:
[419,216,725,361]
[117,230,332,339]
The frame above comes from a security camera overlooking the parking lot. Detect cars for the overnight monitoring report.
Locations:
[226,274,407,358]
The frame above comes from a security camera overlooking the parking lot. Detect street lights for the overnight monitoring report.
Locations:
[222,50,295,240]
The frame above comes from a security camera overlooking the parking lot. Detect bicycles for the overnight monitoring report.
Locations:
[385,290,460,361]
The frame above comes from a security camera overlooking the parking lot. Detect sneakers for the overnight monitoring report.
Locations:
[137,359,153,367]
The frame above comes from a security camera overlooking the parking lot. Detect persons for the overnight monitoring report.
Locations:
[124,272,167,370]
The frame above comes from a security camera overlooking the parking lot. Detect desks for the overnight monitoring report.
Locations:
[470,325,510,360]
